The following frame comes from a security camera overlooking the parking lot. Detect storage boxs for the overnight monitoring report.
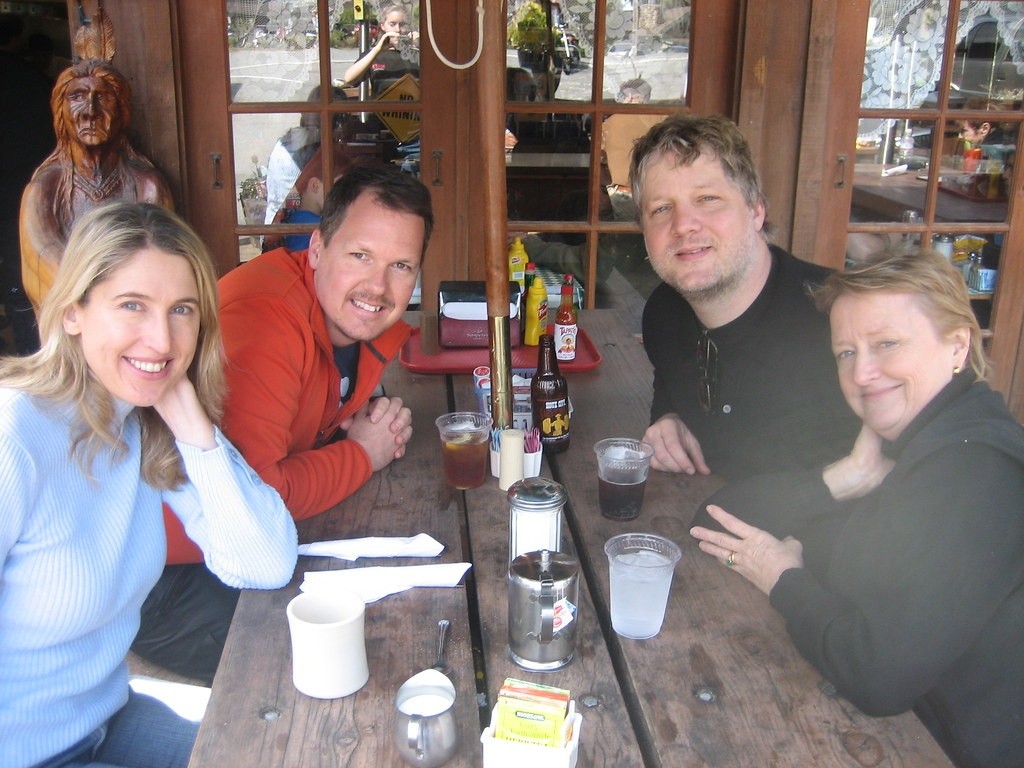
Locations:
[968,268,998,292]
[479,701,583,768]
[489,441,544,480]
[482,392,573,430]
[437,280,522,349]
[473,367,539,413]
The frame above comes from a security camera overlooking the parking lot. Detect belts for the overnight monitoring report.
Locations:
[612,184,632,193]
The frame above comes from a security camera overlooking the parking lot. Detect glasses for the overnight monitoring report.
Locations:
[695,329,719,418]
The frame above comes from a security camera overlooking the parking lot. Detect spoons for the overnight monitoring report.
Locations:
[426,619,452,676]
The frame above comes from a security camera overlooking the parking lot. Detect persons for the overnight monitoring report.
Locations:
[507,186,655,308]
[130,164,436,681]
[0,202,298,768]
[508,56,668,239]
[690,248,1024,768]
[22,61,175,339]
[955,99,1006,146]
[630,117,860,481]
[263,5,420,251]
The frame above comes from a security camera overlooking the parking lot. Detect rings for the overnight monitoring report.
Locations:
[729,551,736,565]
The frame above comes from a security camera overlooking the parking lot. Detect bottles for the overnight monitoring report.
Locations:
[524,278,548,346]
[508,236,529,294]
[903,210,985,287]
[520,262,539,344]
[554,274,578,363]
[508,477,568,590]
[897,128,915,165]
[531,333,569,453]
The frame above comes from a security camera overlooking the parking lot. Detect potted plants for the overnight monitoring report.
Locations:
[505,1,563,73]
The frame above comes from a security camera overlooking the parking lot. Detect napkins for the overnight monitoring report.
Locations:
[299,562,474,605]
[298,532,446,561]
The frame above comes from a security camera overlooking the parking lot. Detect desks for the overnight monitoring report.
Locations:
[851,162,1010,271]
[188,307,959,768]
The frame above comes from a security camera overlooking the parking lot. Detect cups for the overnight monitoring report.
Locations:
[435,411,494,490]
[963,149,982,171]
[594,437,654,522]
[604,533,682,640]
[287,591,370,699]
[509,550,579,669]
[393,668,460,768]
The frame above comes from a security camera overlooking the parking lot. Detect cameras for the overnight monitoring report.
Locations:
[395,36,412,47]
